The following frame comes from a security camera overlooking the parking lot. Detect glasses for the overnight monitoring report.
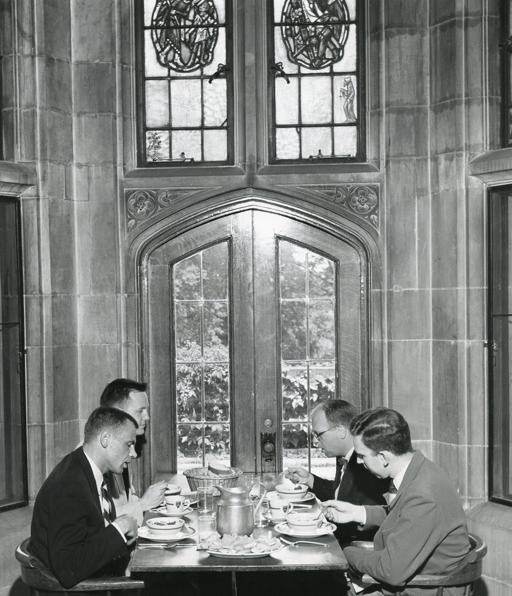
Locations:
[311,425,339,441]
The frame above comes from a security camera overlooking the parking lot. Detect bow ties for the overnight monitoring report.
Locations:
[338,458,348,472]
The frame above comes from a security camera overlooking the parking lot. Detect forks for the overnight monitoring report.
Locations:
[279,536,330,548]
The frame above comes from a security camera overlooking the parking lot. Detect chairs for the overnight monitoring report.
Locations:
[13,536,146,596]
[351,528,486,596]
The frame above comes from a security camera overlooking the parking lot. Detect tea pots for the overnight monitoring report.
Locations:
[214,481,267,541]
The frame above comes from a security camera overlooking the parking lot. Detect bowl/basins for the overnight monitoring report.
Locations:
[146,517,186,535]
[276,483,309,499]
[284,513,318,530]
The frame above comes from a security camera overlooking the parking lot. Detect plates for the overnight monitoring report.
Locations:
[158,506,193,517]
[180,492,201,505]
[274,521,337,538]
[266,491,315,503]
[262,509,294,522]
[137,527,196,544]
[204,550,272,559]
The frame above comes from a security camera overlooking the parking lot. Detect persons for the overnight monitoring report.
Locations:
[28,405,139,590]
[316,405,472,596]
[99,377,167,513]
[283,396,393,549]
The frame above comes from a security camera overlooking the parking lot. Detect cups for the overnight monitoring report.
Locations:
[165,496,191,511]
[158,482,182,506]
[268,500,296,519]
[195,486,214,513]
[196,515,219,546]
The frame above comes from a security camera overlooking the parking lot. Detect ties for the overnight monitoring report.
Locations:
[101,473,112,524]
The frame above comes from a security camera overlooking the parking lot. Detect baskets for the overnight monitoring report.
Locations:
[183,467,243,497]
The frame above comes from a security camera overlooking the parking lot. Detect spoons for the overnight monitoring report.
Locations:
[137,544,178,550]
